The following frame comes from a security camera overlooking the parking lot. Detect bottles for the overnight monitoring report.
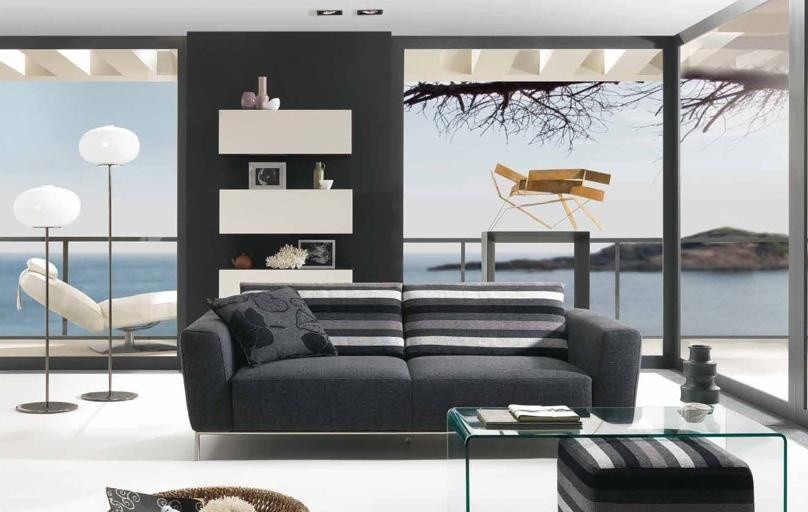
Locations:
[313,162,324,189]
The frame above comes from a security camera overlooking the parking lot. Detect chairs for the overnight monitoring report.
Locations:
[18,260,178,354]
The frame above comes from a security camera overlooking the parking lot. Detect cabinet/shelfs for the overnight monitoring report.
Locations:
[215,108,356,295]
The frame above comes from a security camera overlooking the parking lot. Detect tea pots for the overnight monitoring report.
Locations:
[231,252,252,269]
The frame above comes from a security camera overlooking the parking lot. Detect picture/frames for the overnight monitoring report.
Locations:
[297,238,337,270]
[247,161,287,190]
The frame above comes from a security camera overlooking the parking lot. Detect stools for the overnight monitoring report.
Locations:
[555,435,756,512]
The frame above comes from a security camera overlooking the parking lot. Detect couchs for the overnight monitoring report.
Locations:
[177,279,643,457]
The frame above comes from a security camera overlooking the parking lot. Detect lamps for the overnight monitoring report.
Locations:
[13,125,141,416]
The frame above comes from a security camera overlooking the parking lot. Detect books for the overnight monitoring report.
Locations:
[477,409,584,427]
[508,404,580,422]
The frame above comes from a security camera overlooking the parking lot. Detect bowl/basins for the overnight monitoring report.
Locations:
[319,180,334,189]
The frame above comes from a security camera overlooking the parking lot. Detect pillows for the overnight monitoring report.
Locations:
[204,285,344,370]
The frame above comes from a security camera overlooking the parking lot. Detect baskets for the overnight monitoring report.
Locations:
[151,485,311,512]
